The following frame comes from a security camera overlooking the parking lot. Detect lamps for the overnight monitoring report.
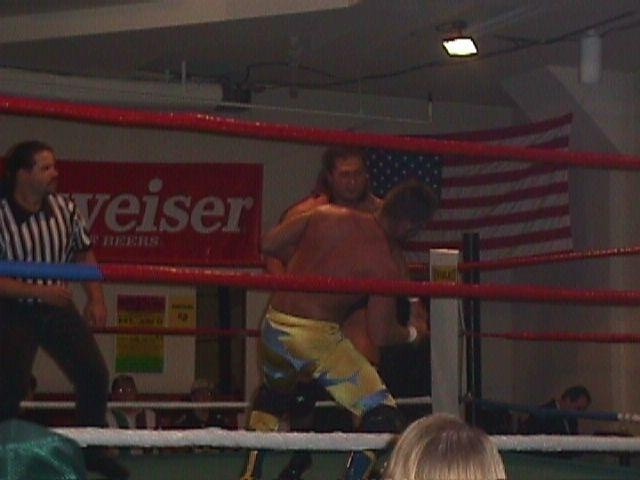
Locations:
[438,16,482,61]
[574,23,606,89]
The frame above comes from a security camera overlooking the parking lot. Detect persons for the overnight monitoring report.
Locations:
[382,412,506,479]
[277,145,428,480]
[1,344,90,479]
[167,377,237,454]
[0,142,131,480]
[515,385,591,459]
[242,179,438,480]
[105,373,188,454]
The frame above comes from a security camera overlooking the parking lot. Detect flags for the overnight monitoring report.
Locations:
[365,114,573,263]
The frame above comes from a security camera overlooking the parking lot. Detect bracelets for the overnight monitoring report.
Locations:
[408,296,420,302]
[407,324,418,343]
